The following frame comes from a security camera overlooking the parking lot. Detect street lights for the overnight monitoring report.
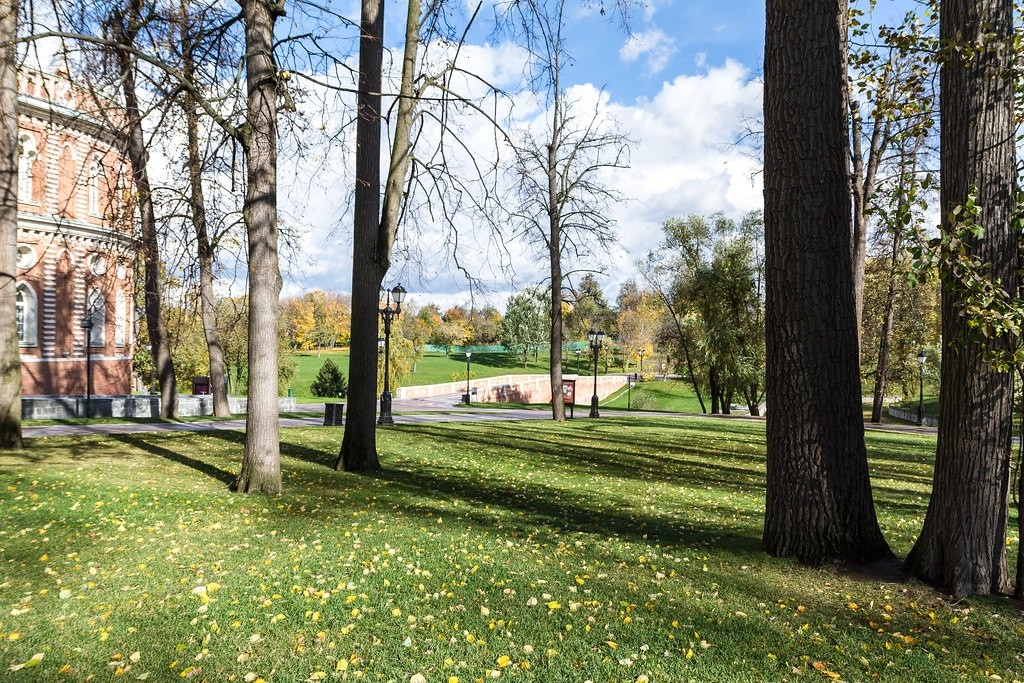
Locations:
[587,328,606,418]
[917,349,927,425]
[576,349,581,376]
[377,283,407,425]
[81,312,95,417]
[638,350,645,371]
[466,353,471,405]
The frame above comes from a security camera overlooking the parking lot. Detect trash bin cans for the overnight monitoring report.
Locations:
[193,376,210,395]
[288,388,294,397]
[462,394,470,405]
[323,403,344,426]
[472,387,477,395]
